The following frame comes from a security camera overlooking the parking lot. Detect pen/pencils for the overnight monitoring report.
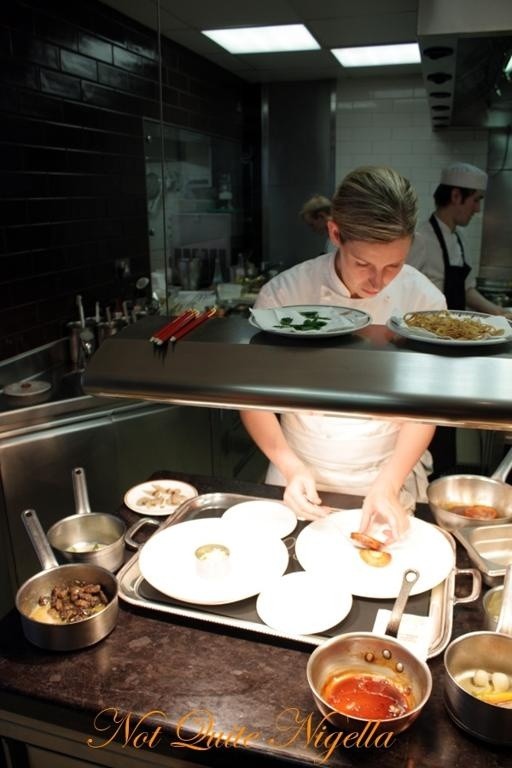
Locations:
[149,307,217,346]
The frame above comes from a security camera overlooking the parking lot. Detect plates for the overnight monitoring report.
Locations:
[295,508,453,600]
[123,480,199,517]
[140,518,290,606]
[5,381,52,397]
[384,310,512,346]
[251,304,374,335]
[221,499,298,541]
[256,569,354,636]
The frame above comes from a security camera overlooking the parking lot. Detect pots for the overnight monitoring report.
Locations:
[426,446,512,533]
[445,630,512,734]
[304,568,435,728]
[16,508,120,652]
[47,467,127,572]
[173,255,208,290]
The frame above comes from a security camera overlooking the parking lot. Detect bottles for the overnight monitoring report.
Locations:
[220,172,233,208]
[212,253,258,289]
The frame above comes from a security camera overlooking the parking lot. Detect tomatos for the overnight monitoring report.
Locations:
[464,503,497,520]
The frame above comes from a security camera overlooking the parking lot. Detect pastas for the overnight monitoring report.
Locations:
[404,314,496,338]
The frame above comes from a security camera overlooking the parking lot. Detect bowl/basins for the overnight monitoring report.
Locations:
[481,581,505,631]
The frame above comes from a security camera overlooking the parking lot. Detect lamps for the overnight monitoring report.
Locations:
[81,302,510,440]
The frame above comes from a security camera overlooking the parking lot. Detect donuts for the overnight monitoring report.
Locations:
[360,551,391,566]
[196,543,229,560]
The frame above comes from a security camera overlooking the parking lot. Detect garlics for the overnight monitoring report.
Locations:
[472,670,488,696]
[489,672,508,695]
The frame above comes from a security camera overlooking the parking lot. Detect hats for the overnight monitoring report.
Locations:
[441,162,488,191]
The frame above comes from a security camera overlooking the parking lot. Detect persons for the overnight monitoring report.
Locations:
[298,190,337,254]
[407,160,511,327]
[235,164,451,549]
